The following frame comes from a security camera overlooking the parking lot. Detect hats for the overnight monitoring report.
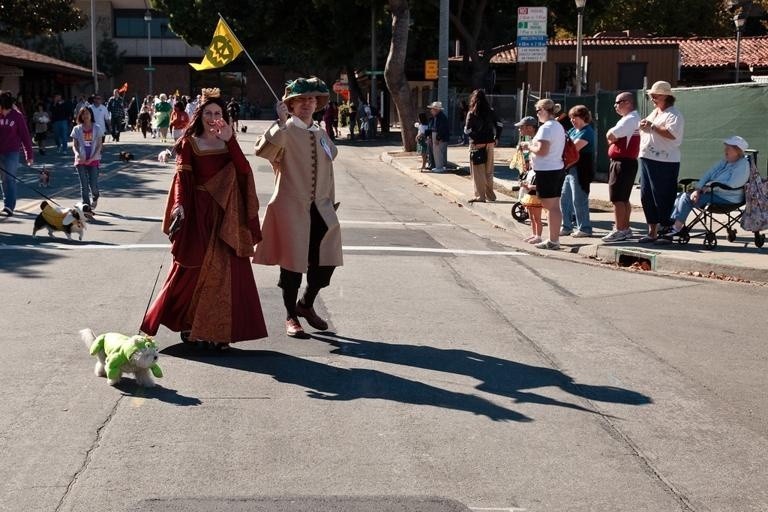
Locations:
[282,77,330,115]
[514,116,538,127]
[427,101,445,110]
[723,136,749,158]
[647,81,673,95]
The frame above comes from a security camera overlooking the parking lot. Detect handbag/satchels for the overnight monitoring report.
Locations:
[741,182,768,231]
[562,137,580,169]
[470,146,487,165]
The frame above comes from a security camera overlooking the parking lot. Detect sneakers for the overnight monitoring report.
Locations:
[285,318,304,338]
[296,300,328,330]
[432,167,446,173]
[523,224,684,250]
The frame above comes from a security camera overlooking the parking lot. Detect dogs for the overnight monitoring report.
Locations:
[158,149,172,164]
[119,151,135,163]
[79,328,159,388]
[241,126,247,133]
[38,163,50,188]
[32,201,95,241]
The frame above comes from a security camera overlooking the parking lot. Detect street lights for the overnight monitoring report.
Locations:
[728,6,748,84]
[142,10,156,96]
[572,0,588,95]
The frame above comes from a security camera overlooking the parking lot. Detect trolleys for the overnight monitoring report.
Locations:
[676,147,766,248]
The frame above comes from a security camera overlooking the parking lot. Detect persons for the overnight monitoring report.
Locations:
[560,105,595,238]
[416,112,430,169]
[638,80,684,246]
[70,106,105,213]
[601,92,641,242]
[518,169,544,245]
[515,116,542,138]
[553,103,572,131]
[5,89,262,156]
[520,98,568,250]
[427,101,449,173]
[0,93,34,217]
[317,96,377,142]
[656,135,750,237]
[251,76,344,339]
[140,96,268,356]
[463,89,503,202]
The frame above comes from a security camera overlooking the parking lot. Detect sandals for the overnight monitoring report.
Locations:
[0,208,13,217]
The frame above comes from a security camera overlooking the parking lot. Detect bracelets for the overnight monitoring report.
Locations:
[650,124,655,132]
